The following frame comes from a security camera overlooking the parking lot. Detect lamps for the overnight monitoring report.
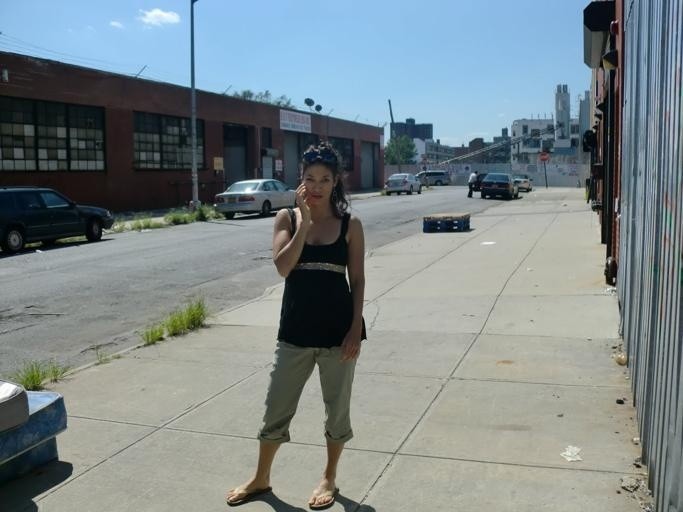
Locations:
[601,51,618,75]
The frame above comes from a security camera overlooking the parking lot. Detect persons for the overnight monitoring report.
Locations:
[467,170,483,199]
[228,139,365,509]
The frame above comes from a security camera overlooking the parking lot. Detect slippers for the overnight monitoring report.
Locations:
[226,485,272,506]
[309,487,339,510]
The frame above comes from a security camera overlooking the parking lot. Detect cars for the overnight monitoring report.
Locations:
[472,173,533,198]
[214,179,297,219]
[386,170,450,195]
[0,186,115,252]
